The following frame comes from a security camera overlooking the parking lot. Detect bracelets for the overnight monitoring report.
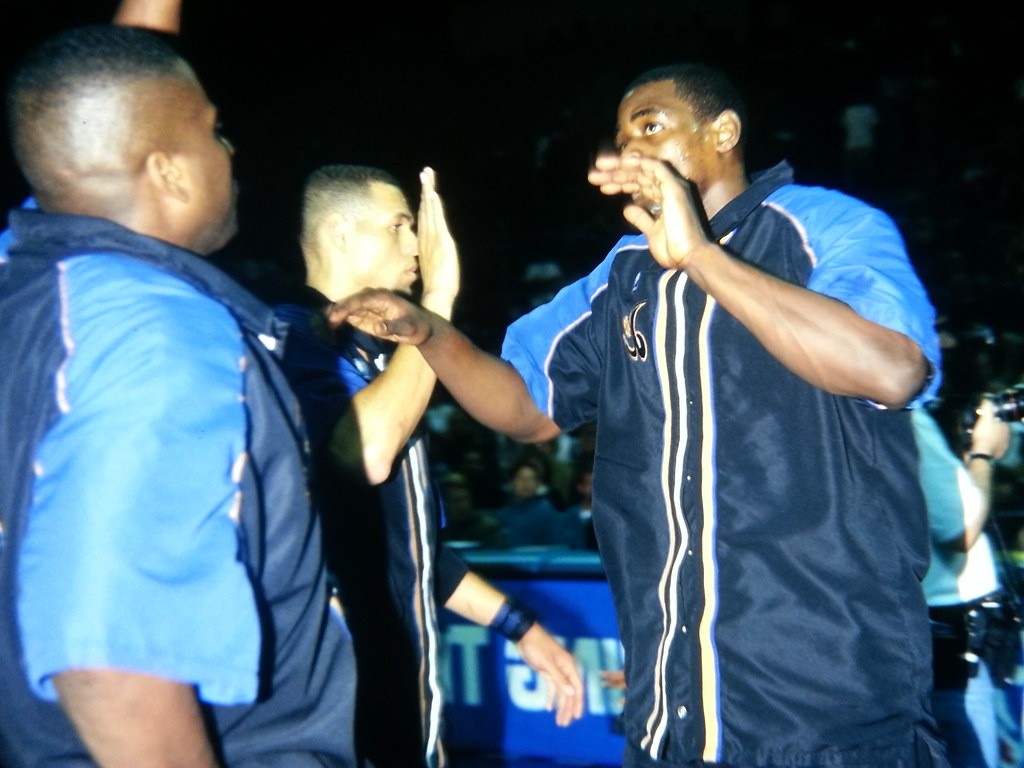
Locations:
[970,453,996,466]
[489,598,535,642]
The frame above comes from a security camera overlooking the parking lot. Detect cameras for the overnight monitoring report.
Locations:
[940,383,1024,453]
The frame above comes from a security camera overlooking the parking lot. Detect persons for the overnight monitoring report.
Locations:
[909,398,1010,689]
[276,163,587,768]
[0,0,358,768]
[934,384,1024,550]
[386,362,600,550]
[328,60,944,768]
